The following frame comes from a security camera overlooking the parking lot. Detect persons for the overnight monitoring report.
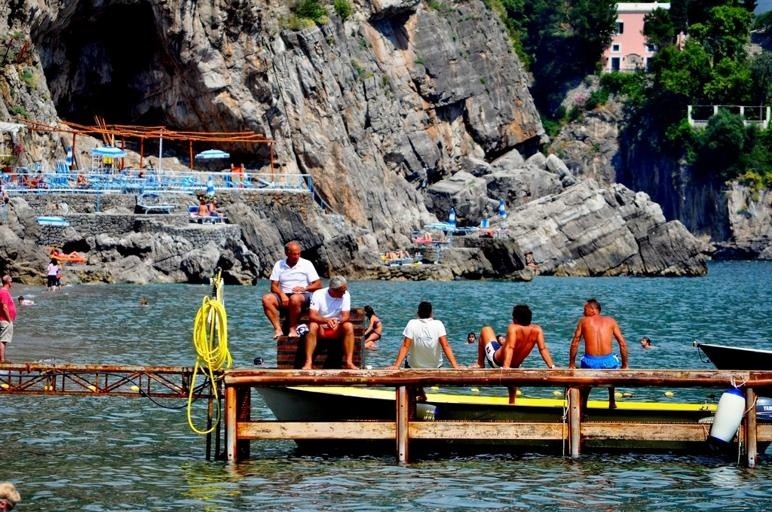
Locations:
[468,304,556,404]
[46,249,88,292]
[569,299,629,409]
[640,337,651,349]
[102,156,112,174]
[0,274,16,364]
[302,275,360,371]
[198,198,224,216]
[363,304,383,349]
[262,240,323,339]
[18,295,34,305]
[386,301,467,370]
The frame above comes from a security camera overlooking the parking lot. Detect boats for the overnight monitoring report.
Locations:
[691,339,772,404]
[248,357,772,457]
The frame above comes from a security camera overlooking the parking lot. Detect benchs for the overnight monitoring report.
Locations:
[274,308,368,373]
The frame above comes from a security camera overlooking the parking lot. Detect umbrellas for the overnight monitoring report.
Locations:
[194,147,230,159]
[91,146,127,159]
[34,213,70,245]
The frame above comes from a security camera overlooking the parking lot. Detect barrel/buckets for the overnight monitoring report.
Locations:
[415,402,437,420]
[481,219,489,228]
